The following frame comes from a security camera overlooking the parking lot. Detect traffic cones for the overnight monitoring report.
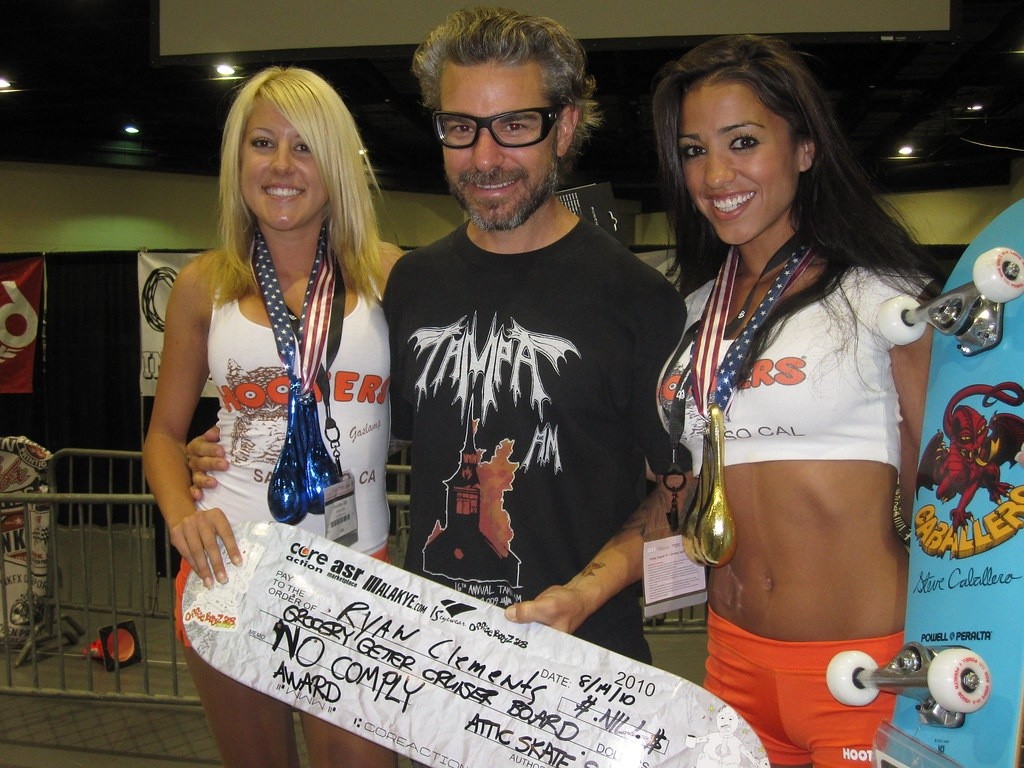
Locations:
[83,619,143,672]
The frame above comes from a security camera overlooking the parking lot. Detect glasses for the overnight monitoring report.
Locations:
[431,104,565,149]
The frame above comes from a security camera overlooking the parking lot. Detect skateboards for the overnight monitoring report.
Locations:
[177,516,773,768]
[813,197,1024,768]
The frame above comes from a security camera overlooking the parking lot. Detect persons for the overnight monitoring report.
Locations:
[143,67,407,768]
[187,6,694,768]
[646,36,944,768]
